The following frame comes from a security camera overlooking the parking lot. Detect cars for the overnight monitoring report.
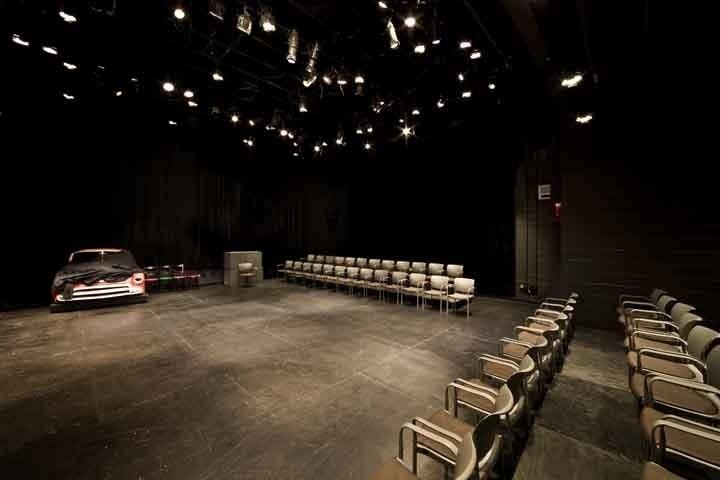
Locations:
[52,247,149,313]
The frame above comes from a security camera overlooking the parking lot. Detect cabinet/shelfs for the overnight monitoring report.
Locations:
[224,251,264,287]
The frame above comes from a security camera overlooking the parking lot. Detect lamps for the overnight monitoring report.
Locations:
[169,1,405,95]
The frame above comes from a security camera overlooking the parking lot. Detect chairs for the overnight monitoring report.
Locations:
[618,290,720,480]
[412,382,513,480]
[487,293,583,410]
[377,423,477,480]
[278,251,481,319]
[235,262,259,287]
[453,354,535,450]
